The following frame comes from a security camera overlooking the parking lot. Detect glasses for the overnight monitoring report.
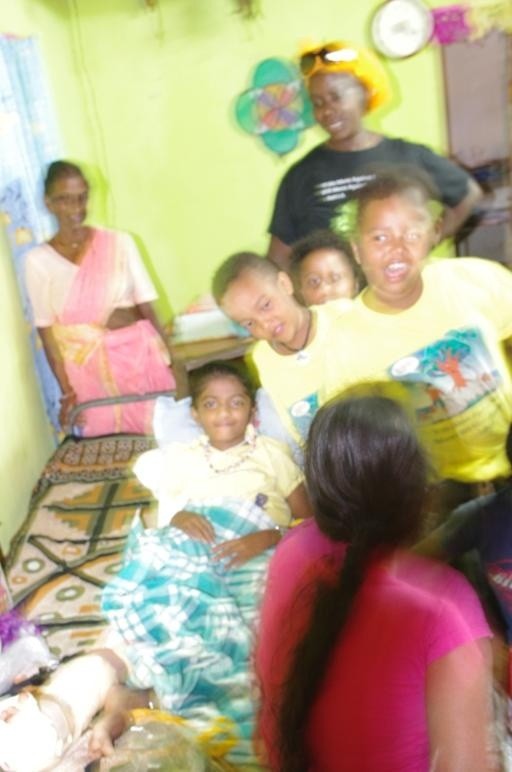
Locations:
[300,45,341,75]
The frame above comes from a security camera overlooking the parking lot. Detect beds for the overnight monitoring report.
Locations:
[0,386,261,772]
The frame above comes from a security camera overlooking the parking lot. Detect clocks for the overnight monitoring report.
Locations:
[368,1,434,60]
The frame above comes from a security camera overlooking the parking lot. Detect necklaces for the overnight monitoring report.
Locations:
[207,439,254,474]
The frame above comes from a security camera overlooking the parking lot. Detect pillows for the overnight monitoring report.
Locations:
[132,384,301,480]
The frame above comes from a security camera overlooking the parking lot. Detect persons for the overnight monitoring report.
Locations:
[290,227,361,306]
[24,158,161,440]
[265,38,485,268]
[213,251,374,444]
[353,170,512,521]
[1,359,314,771]
[256,377,498,771]
